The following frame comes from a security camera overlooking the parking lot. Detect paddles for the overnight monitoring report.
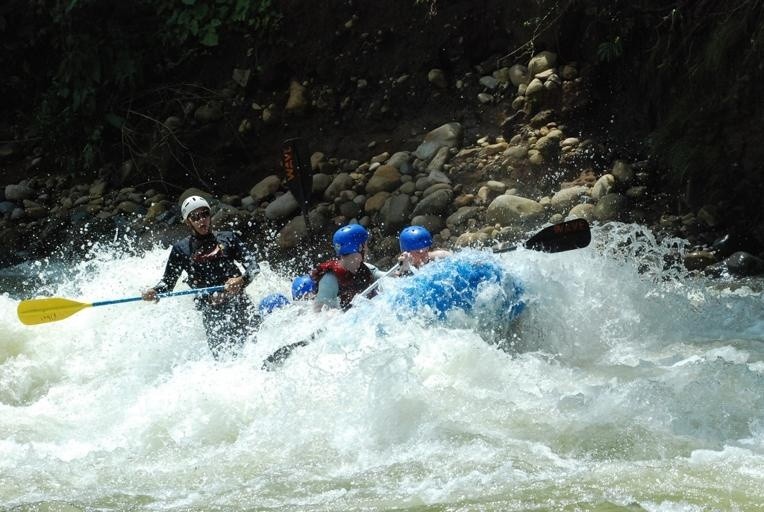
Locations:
[18,286,224,326]
[281,138,319,268]
[261,261,403,372]
[493,218,591,253]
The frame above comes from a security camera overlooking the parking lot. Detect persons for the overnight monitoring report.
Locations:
[142,196,259,358]
[399,225,449,276]
[311,223,414,310]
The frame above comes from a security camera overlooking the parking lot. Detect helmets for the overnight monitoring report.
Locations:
[399,226,433,251]
[333,224,368,255]
[291,274,318,300]
[181,195,211,224]
[259,294,288,314]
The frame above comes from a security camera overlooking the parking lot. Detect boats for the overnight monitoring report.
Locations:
[344,250,531,333]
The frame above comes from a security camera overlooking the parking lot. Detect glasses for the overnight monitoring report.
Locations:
[190,209,210,222]
